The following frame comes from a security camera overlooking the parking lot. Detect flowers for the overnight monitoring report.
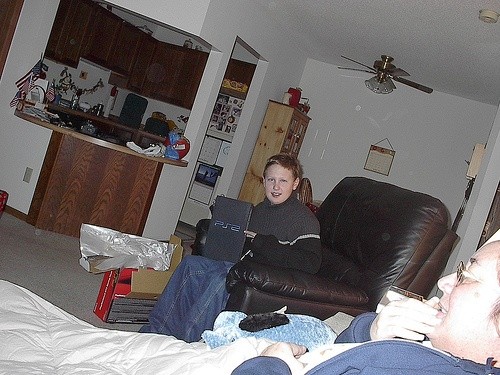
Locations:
[52,67,105,97]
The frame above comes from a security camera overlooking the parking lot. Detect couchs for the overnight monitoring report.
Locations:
[191,176,459,317]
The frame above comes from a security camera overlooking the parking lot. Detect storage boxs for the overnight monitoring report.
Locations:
[94,234,184,325]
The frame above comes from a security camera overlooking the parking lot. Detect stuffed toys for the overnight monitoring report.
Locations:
[202,311,339,350]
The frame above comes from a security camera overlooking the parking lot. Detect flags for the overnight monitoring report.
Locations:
[9,58,56,109]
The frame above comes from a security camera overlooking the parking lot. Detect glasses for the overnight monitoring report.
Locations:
[455,261,487,285]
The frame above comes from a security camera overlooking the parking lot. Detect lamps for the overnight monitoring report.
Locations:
[365,76,396,94]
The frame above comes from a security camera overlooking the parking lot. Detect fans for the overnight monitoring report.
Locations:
[338,55,433,94]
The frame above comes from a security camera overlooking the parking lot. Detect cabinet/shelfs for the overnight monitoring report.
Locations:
[45,0,210,110]
[14,104,189,238]
[237,99,312,206]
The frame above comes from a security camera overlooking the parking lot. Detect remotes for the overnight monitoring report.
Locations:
[239,312,289,332]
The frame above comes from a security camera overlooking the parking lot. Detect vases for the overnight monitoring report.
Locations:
[53,94,63,105]
[71,94,80,110]
[164,133,190,159]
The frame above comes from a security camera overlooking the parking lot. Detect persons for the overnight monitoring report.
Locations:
[136,153,323,343]
[1,240,500,375]
